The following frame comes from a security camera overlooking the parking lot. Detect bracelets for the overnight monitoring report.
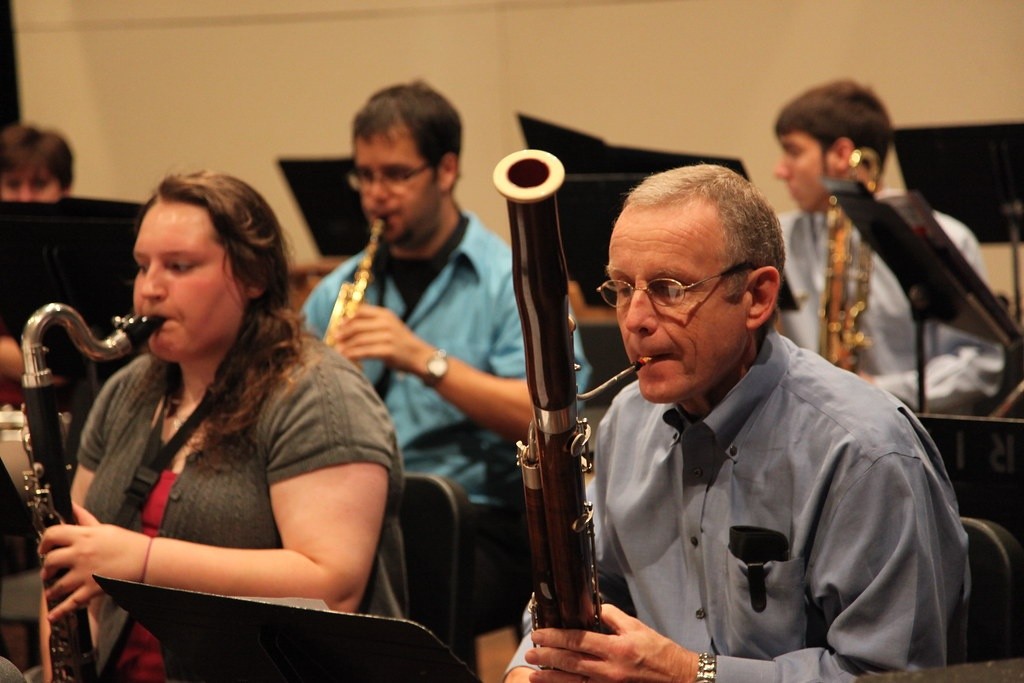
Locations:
[695,651,716,683]
[140,538,154,585]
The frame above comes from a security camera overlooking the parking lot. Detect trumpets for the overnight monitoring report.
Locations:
[812,147,883,373]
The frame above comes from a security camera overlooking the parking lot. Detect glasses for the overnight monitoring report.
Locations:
[595,260,758,307]
[348,160,430,192]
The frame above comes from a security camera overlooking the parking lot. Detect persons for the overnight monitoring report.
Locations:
[39,175,405,683]
[303,81,592,590]
[0,121,76,405]
[502,165,972,683]
[772,77,1004,412]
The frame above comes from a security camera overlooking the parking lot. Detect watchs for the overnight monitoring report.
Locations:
[425,348,449,388]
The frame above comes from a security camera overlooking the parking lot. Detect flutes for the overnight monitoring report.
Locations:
[323,216,392,370]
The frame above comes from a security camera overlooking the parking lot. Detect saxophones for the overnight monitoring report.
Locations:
[15,299,168,683]
